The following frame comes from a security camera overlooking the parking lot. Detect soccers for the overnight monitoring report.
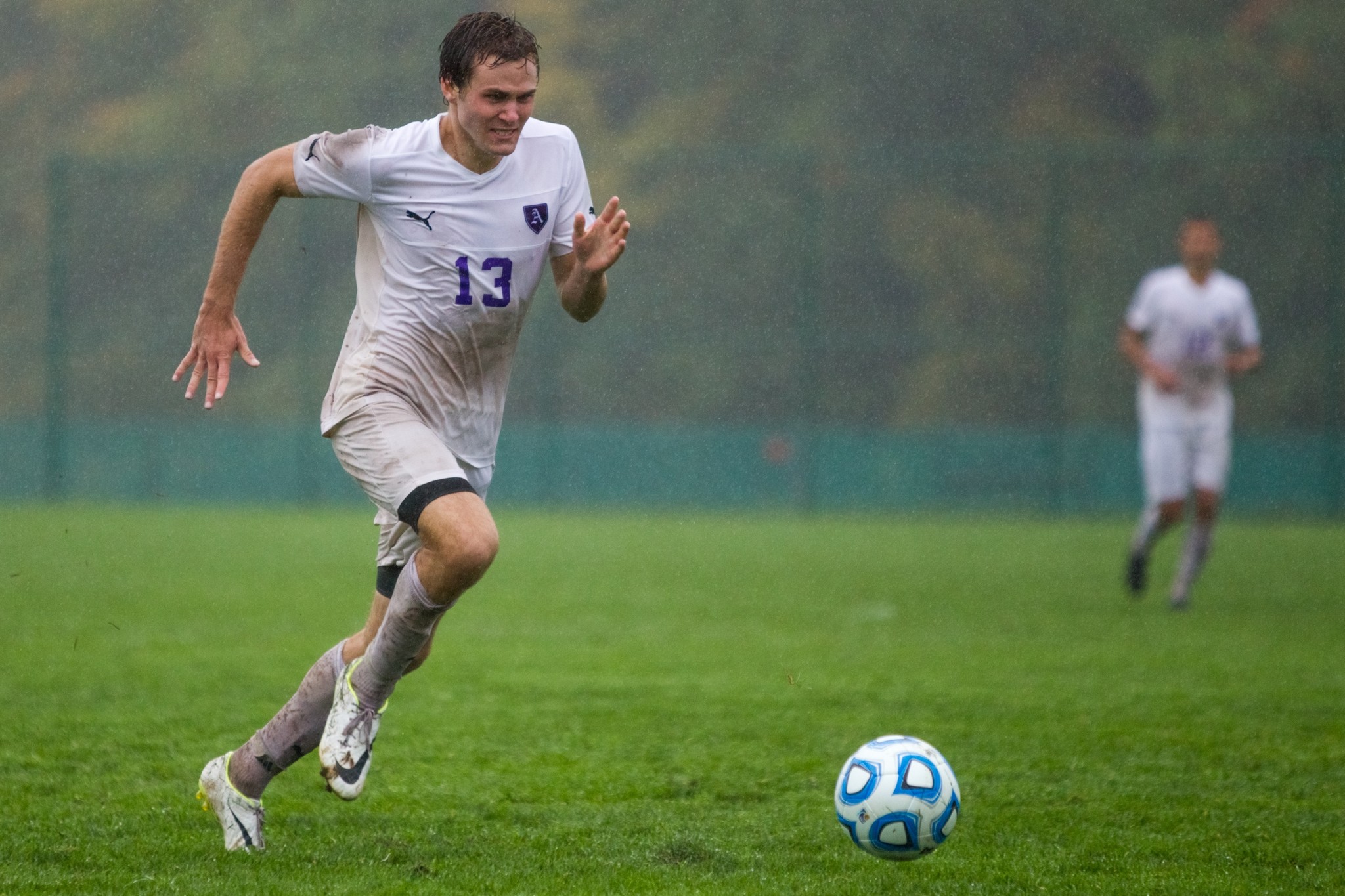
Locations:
[832,732,963,864]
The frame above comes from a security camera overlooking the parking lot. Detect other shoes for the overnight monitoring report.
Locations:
[1124,543,1150,592]
[1168,572,1189,611]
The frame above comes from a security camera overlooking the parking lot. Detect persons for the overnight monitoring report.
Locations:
[168,11,636,862]
[1118,212,1265,608]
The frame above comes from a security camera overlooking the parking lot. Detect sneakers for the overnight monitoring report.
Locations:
[318,655,389,801]
[194,750,266,855]
